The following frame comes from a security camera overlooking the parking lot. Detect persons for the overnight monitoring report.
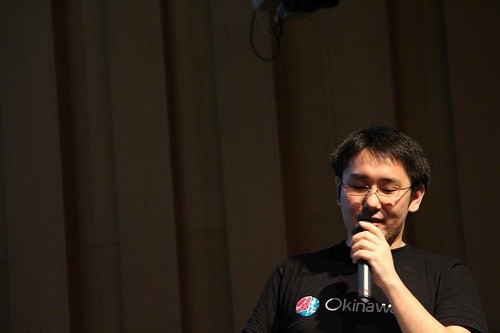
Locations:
[241,125,487,333]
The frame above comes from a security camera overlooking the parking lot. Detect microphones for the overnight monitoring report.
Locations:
[352,226,373,304]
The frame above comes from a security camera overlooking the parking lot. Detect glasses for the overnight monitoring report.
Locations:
[340,182,411,197]
